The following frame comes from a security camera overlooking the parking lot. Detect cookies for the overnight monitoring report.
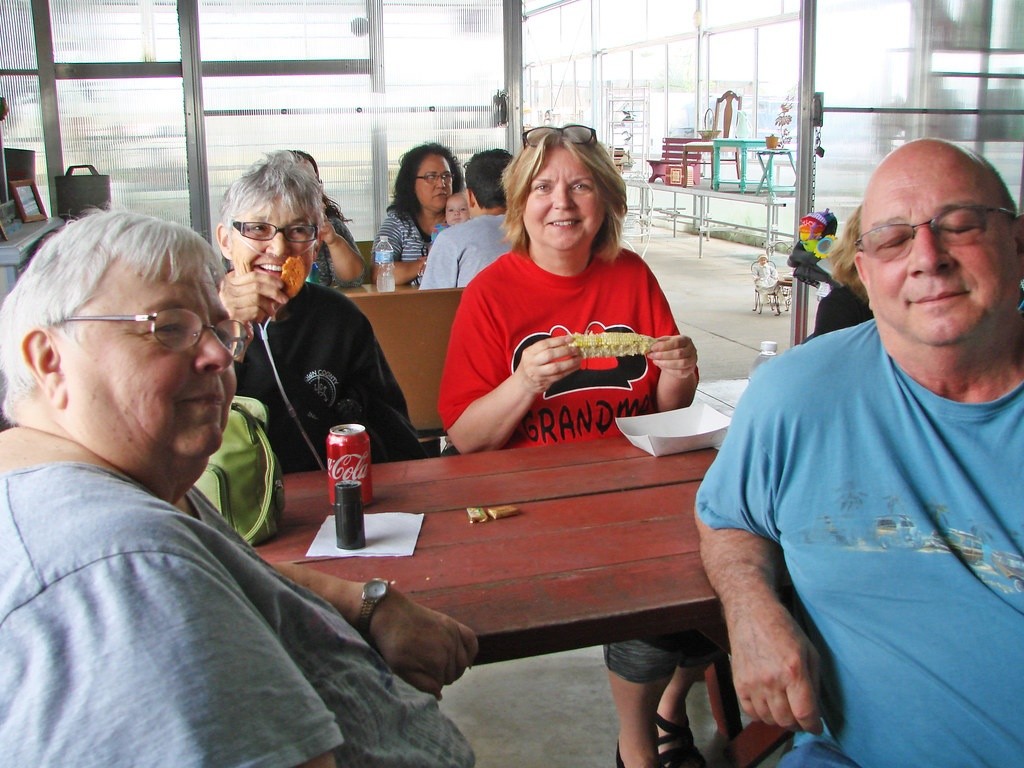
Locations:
[281,256,305,298]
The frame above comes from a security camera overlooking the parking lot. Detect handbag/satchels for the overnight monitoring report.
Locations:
[55,164,112,217]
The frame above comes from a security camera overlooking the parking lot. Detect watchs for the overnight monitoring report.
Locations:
[356,578,389,637]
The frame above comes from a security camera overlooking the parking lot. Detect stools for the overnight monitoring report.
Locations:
[755,147,797,198]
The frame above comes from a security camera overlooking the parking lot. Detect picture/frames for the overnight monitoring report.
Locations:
[8,179,48,223]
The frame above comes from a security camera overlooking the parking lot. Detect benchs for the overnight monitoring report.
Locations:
[348,286,468,435]
[646,137,701,186]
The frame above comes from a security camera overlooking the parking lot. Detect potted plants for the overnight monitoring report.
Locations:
[765,134,779,148]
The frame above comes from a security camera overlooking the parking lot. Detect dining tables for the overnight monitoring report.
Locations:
[255,430,796,768]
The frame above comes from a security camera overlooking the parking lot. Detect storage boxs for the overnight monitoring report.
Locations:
[614,396,732,456]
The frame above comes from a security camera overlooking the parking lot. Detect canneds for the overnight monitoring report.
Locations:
[326,423,373,506]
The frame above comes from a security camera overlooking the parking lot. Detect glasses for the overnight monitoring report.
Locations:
[855,203,1017,263]
[418,173,458,187]
[521,125,598,151]
[231,217,318,244]
[52,310,253,363]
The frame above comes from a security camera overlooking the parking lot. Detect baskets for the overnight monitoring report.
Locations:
[696,108,721,141]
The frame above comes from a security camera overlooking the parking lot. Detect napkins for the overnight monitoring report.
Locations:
[305,512,426,558]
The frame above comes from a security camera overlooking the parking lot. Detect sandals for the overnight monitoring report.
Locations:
[652,711,706,768]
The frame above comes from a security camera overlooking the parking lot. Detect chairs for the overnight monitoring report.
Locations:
[752,261,781,317]
[683,90,743,189]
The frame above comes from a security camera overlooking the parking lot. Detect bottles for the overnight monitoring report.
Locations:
[748,341,778,379]
[375,236,396,293]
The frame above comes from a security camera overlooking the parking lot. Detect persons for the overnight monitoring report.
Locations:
[285,150,370,291]
[430,192,470,243]
[803,200,875,345]
[370,143,465,287]
[0,208,480,768]
[205,148,428,474]
[418,148,513,291]
[438,126,731,768]
[692,136,1024,768]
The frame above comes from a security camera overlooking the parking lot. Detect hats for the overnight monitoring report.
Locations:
[756,254,769,260]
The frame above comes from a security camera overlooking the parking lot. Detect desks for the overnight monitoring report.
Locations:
[711,138,773,195]
[640,178,796,262]
[779,280,792,311]
[622,178,643,240]
[338,281,419,296]
